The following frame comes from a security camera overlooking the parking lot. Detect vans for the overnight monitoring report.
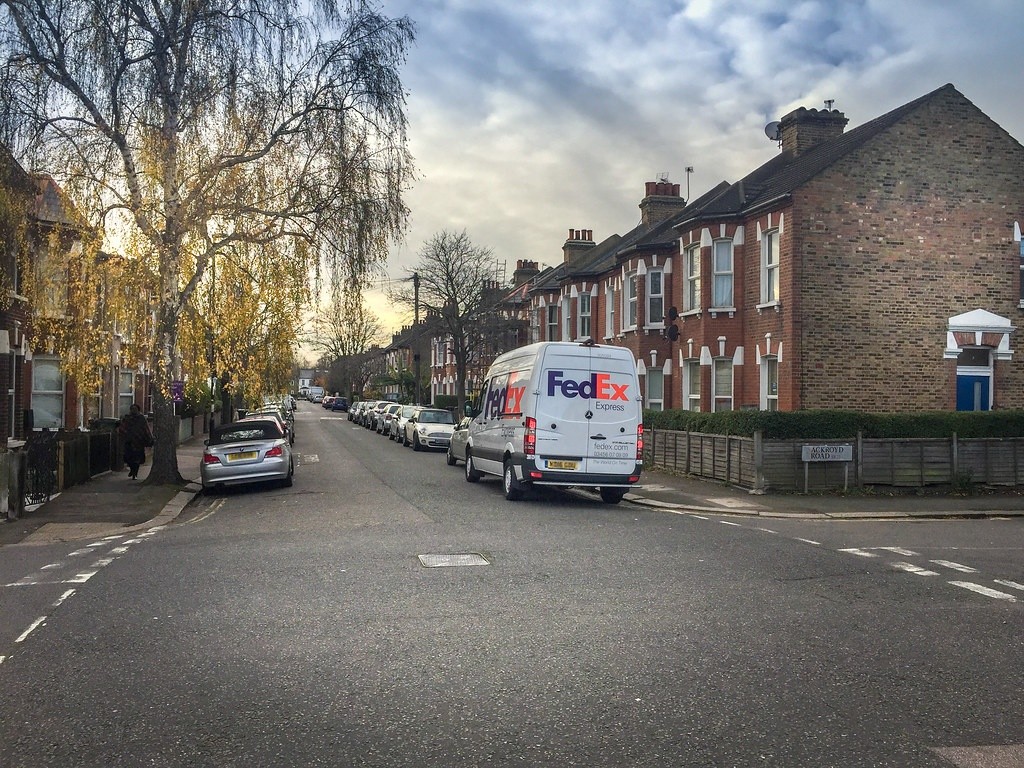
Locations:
[465,336,644,504]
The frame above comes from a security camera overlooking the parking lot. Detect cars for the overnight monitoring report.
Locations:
[305,386,474,466]
[237,393,297,447]
[200,416,294,495]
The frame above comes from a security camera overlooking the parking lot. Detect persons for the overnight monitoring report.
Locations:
[120,404,151,480]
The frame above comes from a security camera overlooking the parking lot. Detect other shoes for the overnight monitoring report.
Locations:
[133,474,137,479]
[128,470,133,477]
